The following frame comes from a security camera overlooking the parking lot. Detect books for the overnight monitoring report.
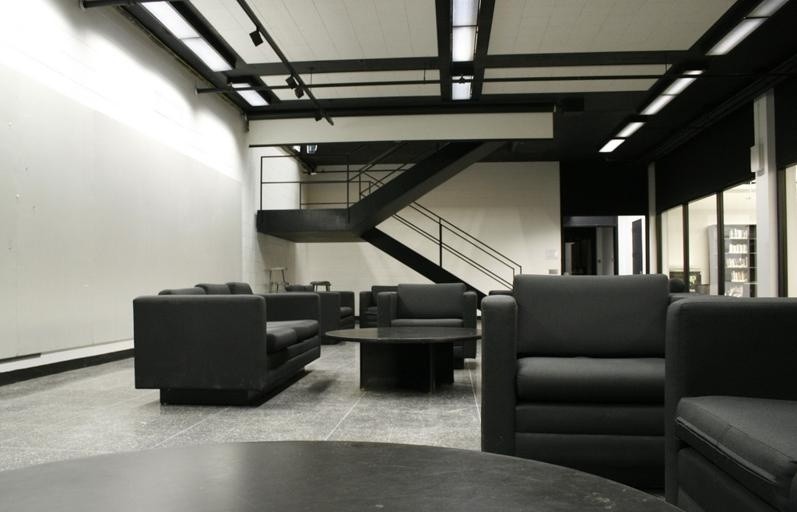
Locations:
[724,228,749,298]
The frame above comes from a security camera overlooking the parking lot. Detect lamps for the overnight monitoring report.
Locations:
[237,0,335,128]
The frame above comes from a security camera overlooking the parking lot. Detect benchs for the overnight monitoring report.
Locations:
[133,282,321,407]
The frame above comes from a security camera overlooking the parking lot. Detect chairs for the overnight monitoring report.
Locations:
[359,285,397,328]
[664,297,796,511]
[377,284,477,369]
[481,273,669,490]
[316,291,355,345]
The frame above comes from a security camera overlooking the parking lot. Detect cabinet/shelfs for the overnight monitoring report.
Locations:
[707,223,758,298]
